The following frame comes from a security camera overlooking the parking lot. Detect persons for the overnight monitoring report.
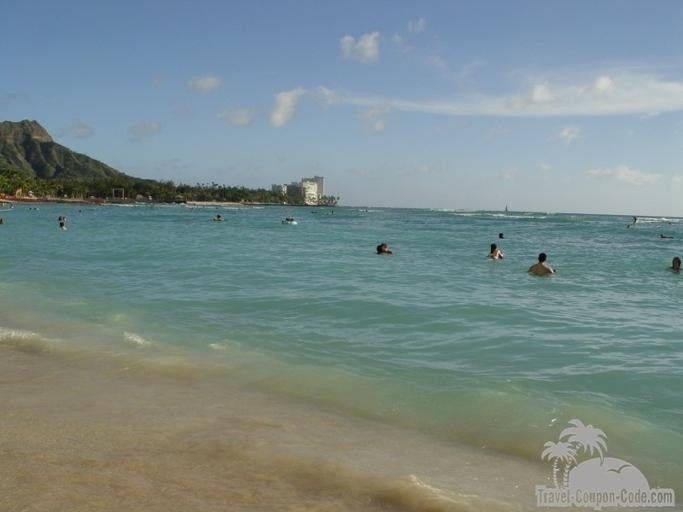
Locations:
[499,232,504,239]
[488,243,503,260]
[58,222,67,231]
[213,214,224,222]
[528,252,556,276]
[376,243,392,254]
[666,256,683,273]
[289,218,297,225]
